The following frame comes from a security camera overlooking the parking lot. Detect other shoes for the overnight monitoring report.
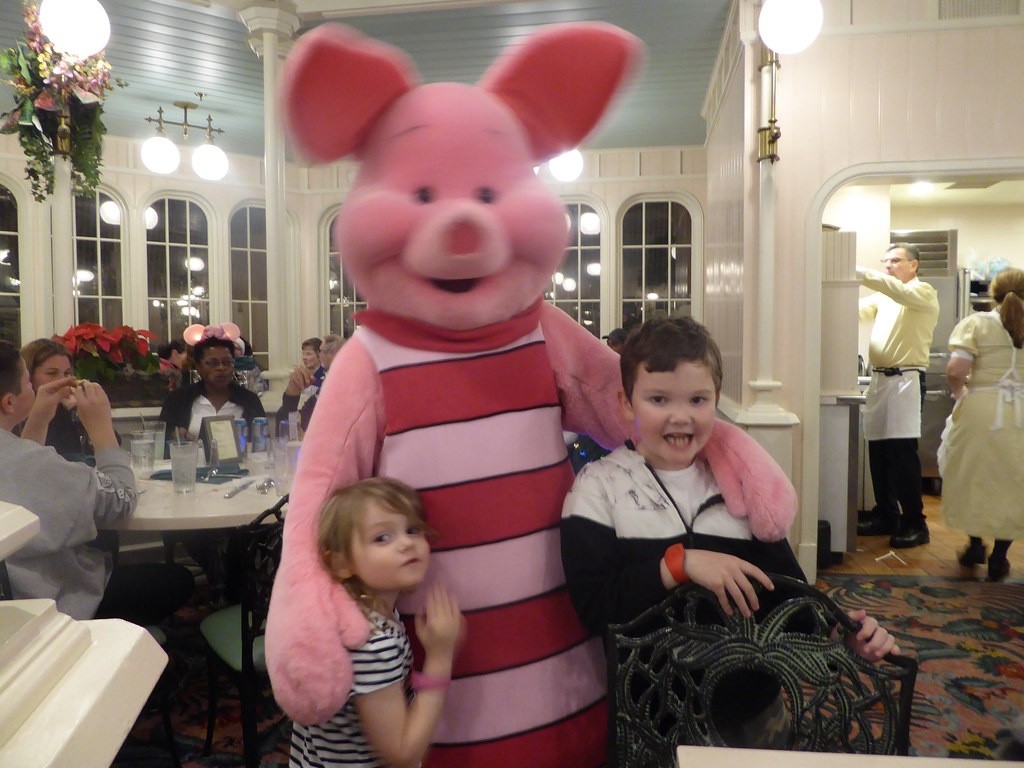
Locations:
[987,553,1010,579]
[959,543,985,565]
[889,522,929,549]
[857,511,899,535]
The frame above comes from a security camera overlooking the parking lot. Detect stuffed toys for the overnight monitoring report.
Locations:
[264,21,802,768]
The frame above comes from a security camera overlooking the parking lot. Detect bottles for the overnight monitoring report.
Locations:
[210,439,220,469]
[197,439,206,468]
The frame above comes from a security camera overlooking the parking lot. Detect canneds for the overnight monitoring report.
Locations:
[279,420,299,442]
[251,417,270,452]
[231,418,248,457]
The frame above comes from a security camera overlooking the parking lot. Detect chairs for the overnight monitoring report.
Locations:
[99,492,290,768]
[603,570,918,768]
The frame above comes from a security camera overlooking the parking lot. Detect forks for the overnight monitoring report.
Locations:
[256,483,264,490]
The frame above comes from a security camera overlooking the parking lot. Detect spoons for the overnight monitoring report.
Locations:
[204,469,216,483]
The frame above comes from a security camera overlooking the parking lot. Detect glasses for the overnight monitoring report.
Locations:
[198,357,236,368]
[881,258,912,265]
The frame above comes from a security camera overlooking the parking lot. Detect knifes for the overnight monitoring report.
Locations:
[224,480,255,498]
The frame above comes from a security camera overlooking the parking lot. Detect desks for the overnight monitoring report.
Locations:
[99,441,302,641]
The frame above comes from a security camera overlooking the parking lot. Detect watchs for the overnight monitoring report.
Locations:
[665,543,689,585]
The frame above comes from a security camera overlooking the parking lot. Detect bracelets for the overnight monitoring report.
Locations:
[412,671,451,692]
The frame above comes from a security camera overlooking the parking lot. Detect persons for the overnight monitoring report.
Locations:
[285,477,468,768]
[937,267,1024,580]
[852,243,940,549]
[0,322,345,720]
[600,328,628,356]
[559,317,902,768]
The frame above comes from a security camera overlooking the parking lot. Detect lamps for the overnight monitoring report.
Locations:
[141,101,230,181]
[39,0,111,162]
[757,0,825,163]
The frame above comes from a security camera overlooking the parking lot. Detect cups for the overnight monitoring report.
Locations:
[253,383,264,398]
[130,430,156,479]
[289,412,301,440]
[190,370,202,384]
[266,437,288,469]
[61,393,77,411]
[143,421,166,460]
[275,441,303,495]
[79,435,96,468]
[169,441,198,494]
[279,420,288,437]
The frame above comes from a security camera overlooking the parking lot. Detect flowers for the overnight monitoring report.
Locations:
[0,0,114,207]
[52,320,179,407]
